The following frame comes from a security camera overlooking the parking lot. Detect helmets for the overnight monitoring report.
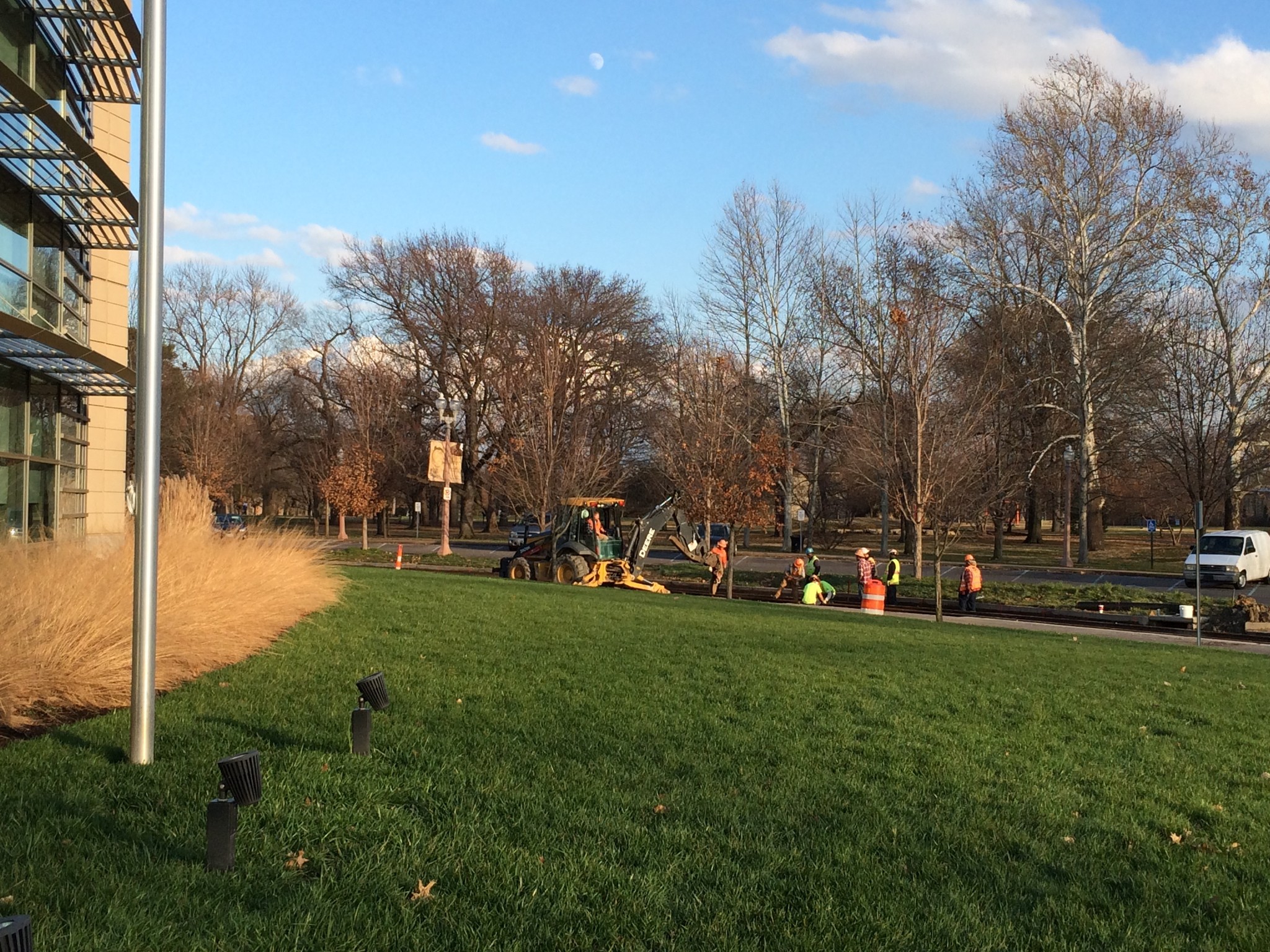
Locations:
[860,547,870,554]
[717,539,726,548]
[806,547,814,554]
[888,549,899,555]
[854,549,865,557]
[964,554,975,562]
[793,559,804,568]
[809,574,820,583]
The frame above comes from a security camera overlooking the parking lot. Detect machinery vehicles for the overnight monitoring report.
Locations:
[490,493,721,602]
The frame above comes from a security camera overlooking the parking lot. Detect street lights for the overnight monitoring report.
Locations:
[1060,443,1075,566]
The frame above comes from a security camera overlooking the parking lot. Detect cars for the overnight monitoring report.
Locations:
[3,520,24,543]
[211,513,249,540]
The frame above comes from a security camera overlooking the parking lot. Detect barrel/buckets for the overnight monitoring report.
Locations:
[1179,604,1193,619]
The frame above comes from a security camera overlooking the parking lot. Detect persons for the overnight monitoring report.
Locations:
[802,574,824,606]
[586,513,608,549]
[771,559,805,601]
[882,549,900,606]
[707,540,728,596]
[958,554,983,612]
[816,579,835,604]
[804,547,820,581]
[861,547,878,579]
[848,549,872,601]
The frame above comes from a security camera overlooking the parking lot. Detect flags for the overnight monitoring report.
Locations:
[448,442,463,484]
[427,439,446,482]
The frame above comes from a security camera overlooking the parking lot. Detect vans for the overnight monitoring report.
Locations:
[1182,528,1269,588]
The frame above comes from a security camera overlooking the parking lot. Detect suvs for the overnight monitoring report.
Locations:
[508,510,555,550]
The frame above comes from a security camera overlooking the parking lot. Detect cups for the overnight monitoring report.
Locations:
[1099,605,1104,613]
